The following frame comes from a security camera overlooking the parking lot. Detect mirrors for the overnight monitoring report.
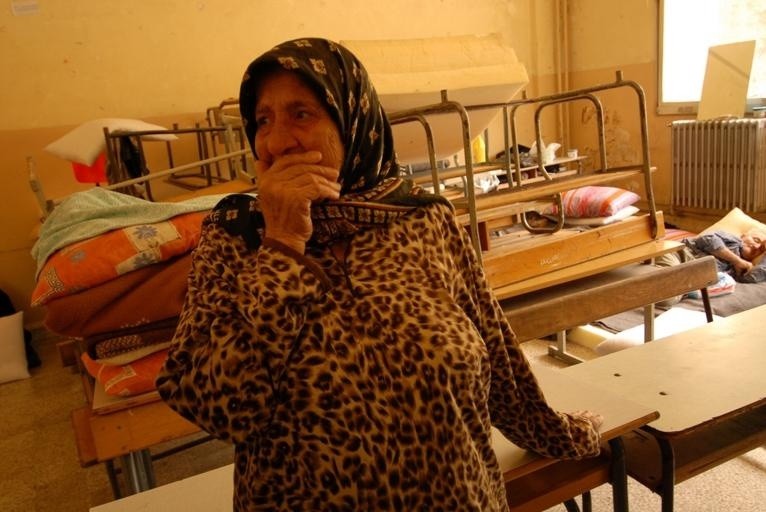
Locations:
[697,40,755,120]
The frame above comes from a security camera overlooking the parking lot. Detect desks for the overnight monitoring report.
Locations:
[29,69,766,512]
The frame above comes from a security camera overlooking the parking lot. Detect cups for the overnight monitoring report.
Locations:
[752,107,766,118]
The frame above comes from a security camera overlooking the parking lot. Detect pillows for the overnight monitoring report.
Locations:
[0,311,32,384]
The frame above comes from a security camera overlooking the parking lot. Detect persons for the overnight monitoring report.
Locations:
[644,230,766,307]
[156,39,604,512]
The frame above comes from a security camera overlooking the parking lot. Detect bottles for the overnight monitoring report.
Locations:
[567,148,577,158]
[472,134,487,163]
